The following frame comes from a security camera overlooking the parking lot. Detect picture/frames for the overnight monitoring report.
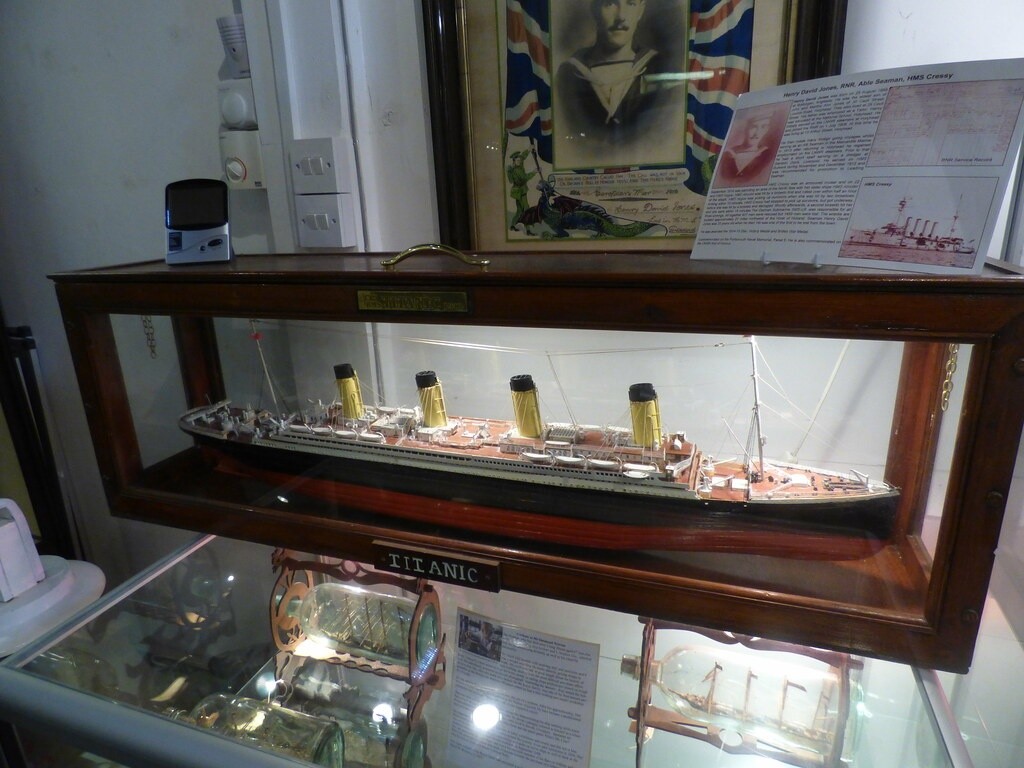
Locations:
[422,0,847,255]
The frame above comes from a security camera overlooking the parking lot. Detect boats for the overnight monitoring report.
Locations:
[847,193,976,254]
[172,318,904,563]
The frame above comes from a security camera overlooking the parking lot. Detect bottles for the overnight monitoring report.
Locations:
[619,635,867,759]
[268,583,437,666]
[277,696,424,768]
[160,691,345,768]
